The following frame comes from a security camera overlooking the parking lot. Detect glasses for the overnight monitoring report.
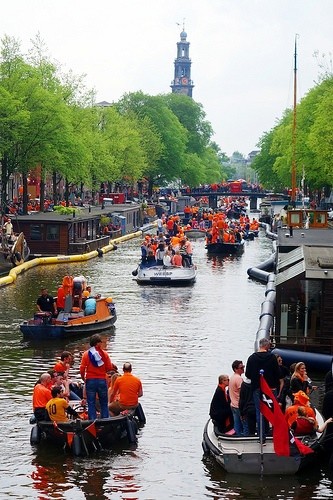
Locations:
[54,376,57,377]
[278,359,282,363]
[223,379,229,382]
[238,365,245,369]
[60,390,63,393]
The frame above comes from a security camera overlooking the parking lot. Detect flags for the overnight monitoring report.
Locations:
[63,426,102,445]
[256,366,314,457]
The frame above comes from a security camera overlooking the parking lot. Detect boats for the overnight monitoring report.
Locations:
[146,248,193,265]
[18,295,118,339]
[204,236,245,257]
[181,216,259,240]
[131,260,198,287]
[202,408,309,477]
[29,378,145,457]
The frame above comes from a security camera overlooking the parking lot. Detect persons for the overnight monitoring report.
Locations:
[0,176,333,270]
[210,337,317,436]
[32,274,100,316]
[31,333,145,425]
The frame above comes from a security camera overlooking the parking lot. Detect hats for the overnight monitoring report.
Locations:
[292,390,309,407]
